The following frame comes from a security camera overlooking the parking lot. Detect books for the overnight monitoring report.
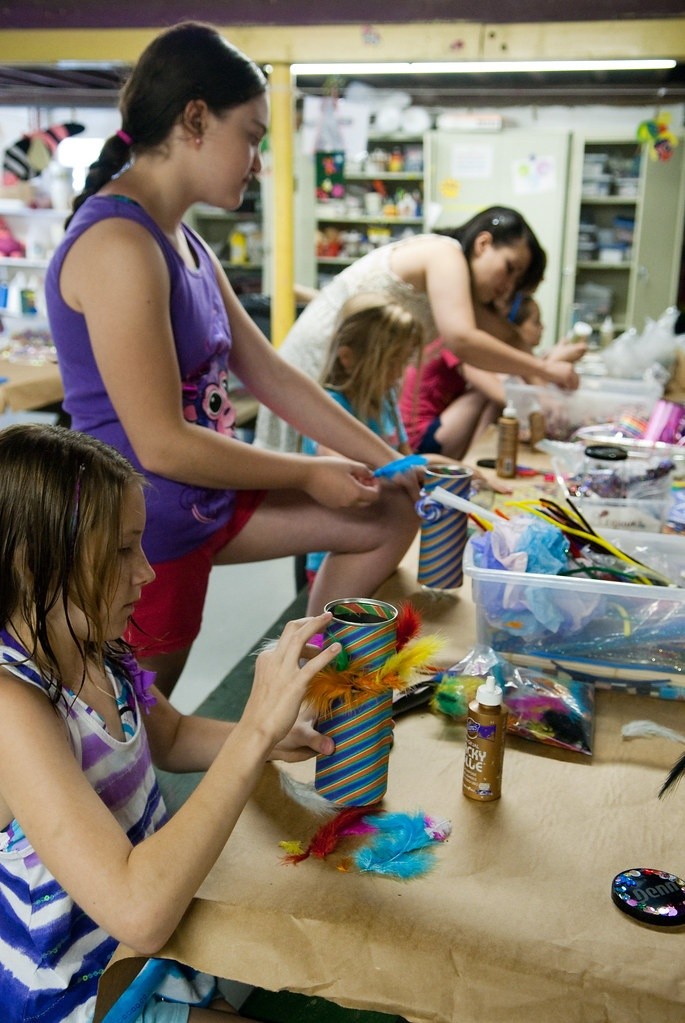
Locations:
[576,147,640,267]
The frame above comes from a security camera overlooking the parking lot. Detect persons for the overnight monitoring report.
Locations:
[295,301,429,602]
[248,203,581,479]
[498,288,587,365]
[396,328,502,465]
[45,19,432,733]
[2,421,342,1022]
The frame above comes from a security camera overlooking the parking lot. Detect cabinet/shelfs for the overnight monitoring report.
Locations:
[1,64,272,431]
[290,64,685,355]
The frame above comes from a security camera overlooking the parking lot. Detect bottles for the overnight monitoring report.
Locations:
[465,673,505,803]
[528,400,547,446]
[495,400,519,477]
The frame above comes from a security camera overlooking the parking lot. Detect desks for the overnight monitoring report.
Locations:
[89,423,684,1022]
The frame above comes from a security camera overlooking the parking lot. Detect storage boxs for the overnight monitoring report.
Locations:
[459,514,685,696]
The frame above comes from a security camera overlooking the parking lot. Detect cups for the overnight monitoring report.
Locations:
[583,444,627,498]
